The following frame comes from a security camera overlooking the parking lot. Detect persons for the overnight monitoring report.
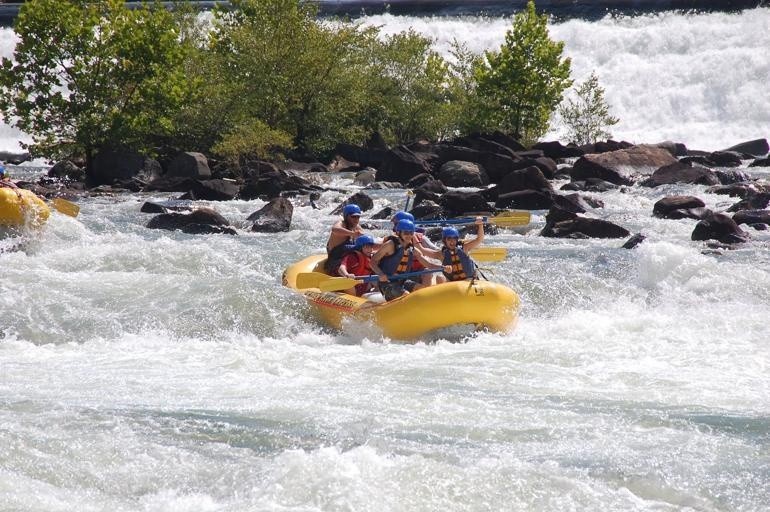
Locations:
[368,219,452,303]
[388,211,447,287]
[0,165,13,187]
[411,217,484,284]
[327,204,367,259]
[325,236,381,298]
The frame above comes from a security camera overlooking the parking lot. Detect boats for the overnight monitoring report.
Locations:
[0,189,54,230]
[282,252,521,347]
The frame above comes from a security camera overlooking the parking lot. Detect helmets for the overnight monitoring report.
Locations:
[344,204,361,219]
[393,212,415,233]
[441,229,458,240]
[357,235,373,249]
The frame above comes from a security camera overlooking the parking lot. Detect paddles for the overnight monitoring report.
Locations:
[319,266,456,293]
[469,248,508,263]
[297,272,397,286]
[416,213,530,229]
